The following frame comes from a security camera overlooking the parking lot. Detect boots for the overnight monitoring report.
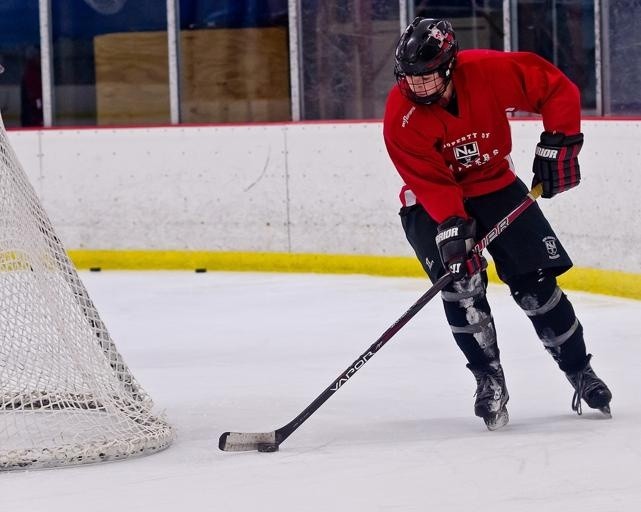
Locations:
[465,358,509,417]
[564,353,610,416]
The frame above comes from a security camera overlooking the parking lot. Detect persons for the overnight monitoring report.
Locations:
[383,16,613,430]
[19,55,44,128]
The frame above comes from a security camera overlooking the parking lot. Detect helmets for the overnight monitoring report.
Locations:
[393,17,459,105]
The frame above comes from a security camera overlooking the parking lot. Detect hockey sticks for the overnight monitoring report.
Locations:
[218,184,544,451]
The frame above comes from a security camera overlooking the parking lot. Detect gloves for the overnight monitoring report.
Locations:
[530,130,584,199]
[435,216,489,282]
